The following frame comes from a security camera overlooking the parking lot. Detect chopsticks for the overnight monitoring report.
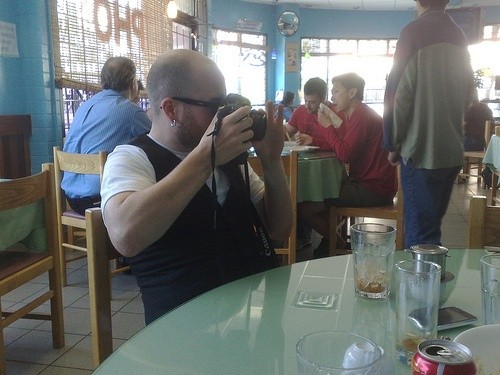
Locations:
[285,131,292,144]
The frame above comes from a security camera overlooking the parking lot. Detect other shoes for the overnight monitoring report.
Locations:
[313,231,346,256]
[297,240,312,250]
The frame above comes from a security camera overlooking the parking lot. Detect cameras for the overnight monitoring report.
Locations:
[225,93,267,165]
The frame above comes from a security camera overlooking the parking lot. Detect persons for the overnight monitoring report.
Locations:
[276,92,295,121]
[100,49,293,325]
[284,77,345,251]
[382,1,473,250]
[298,72,398,254]
[60,56,152,275]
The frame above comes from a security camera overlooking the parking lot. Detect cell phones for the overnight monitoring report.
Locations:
[411,305,480,332]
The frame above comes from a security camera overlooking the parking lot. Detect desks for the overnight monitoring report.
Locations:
[92,249,500,375]
[247,140,345,203]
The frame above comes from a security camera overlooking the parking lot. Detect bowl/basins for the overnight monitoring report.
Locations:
[453,324,500,375]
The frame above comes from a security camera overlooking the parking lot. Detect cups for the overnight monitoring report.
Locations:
[480,254,500,325]
[295,328,382,375]
[394,259,442,368]
[409,243,449,281]
[350,222,397,299]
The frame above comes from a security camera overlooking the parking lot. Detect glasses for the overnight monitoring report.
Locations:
[160,96,223,110]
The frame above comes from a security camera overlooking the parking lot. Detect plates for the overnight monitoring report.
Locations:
[283,146,320,152]
[284,141,301,146]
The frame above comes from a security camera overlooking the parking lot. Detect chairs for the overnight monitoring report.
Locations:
[0,120,500,375]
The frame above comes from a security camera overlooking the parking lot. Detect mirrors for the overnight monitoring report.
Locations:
[277,10,300,37]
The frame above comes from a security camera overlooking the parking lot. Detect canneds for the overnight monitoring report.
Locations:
[411,339,476,375]
